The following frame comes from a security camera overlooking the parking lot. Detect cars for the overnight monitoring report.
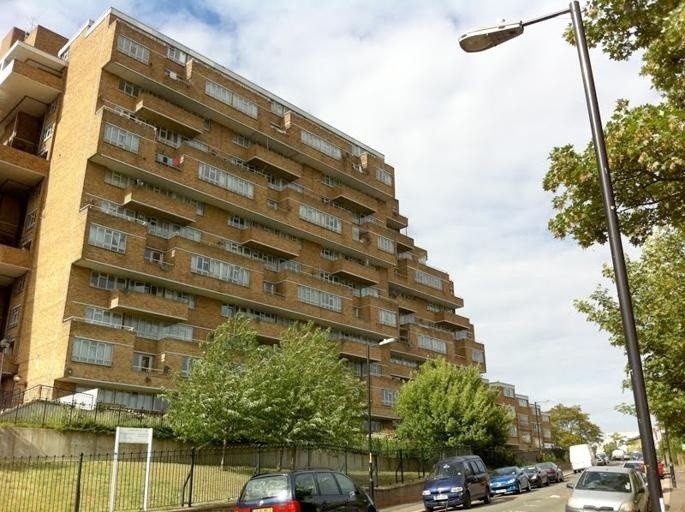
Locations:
[488,459,566,497]
[563,437,667,511]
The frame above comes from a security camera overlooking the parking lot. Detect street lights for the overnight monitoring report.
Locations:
[365,335,400,504]
[534,399,550,461]
[452,1,668,511]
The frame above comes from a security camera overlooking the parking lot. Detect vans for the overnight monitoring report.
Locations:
[232,464,378,511]
[420,454,493,511]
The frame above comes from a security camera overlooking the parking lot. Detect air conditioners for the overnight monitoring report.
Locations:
[158,153,172,166]
